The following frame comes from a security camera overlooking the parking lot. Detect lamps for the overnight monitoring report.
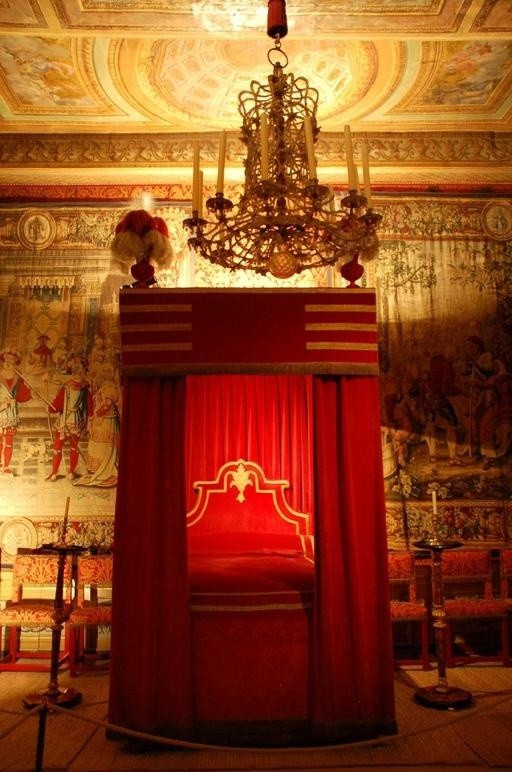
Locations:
[183,1,384,279]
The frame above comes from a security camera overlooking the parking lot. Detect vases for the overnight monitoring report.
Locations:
[340,252,365,289]
[132,253,156,290]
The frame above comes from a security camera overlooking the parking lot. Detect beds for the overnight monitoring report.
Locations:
[103,282,403,750]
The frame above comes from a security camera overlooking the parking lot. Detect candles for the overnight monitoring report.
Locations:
[64,496,70,519]
[431,490,438,514]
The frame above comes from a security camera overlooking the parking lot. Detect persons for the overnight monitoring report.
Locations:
[12,40,77,106]
[441,43,493,105]
[22,278,69,301]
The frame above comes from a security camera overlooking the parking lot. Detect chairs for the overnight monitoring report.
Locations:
[67,556,114,677]
[0,555,73,671]
[388,549,429,671]
[431,546,512,668]
